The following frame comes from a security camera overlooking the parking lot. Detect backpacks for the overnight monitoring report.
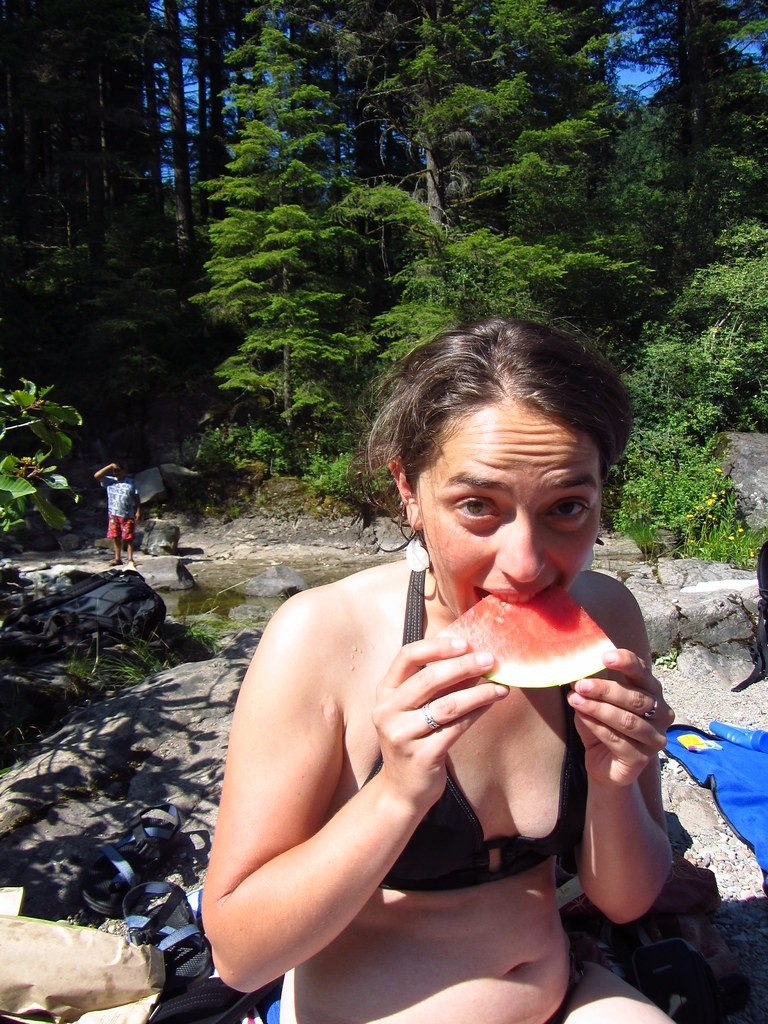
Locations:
[750,540,768,678]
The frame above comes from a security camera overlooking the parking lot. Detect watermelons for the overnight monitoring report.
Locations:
[424,583,621,691]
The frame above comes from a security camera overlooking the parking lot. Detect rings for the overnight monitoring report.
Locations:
[423,703,439,729]
[645,699,658,718]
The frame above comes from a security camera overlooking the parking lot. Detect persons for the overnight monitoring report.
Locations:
[94,463,141,566]
[201,311,675,1023]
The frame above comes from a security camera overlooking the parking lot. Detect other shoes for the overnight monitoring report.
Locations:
[110,560,122,566]
